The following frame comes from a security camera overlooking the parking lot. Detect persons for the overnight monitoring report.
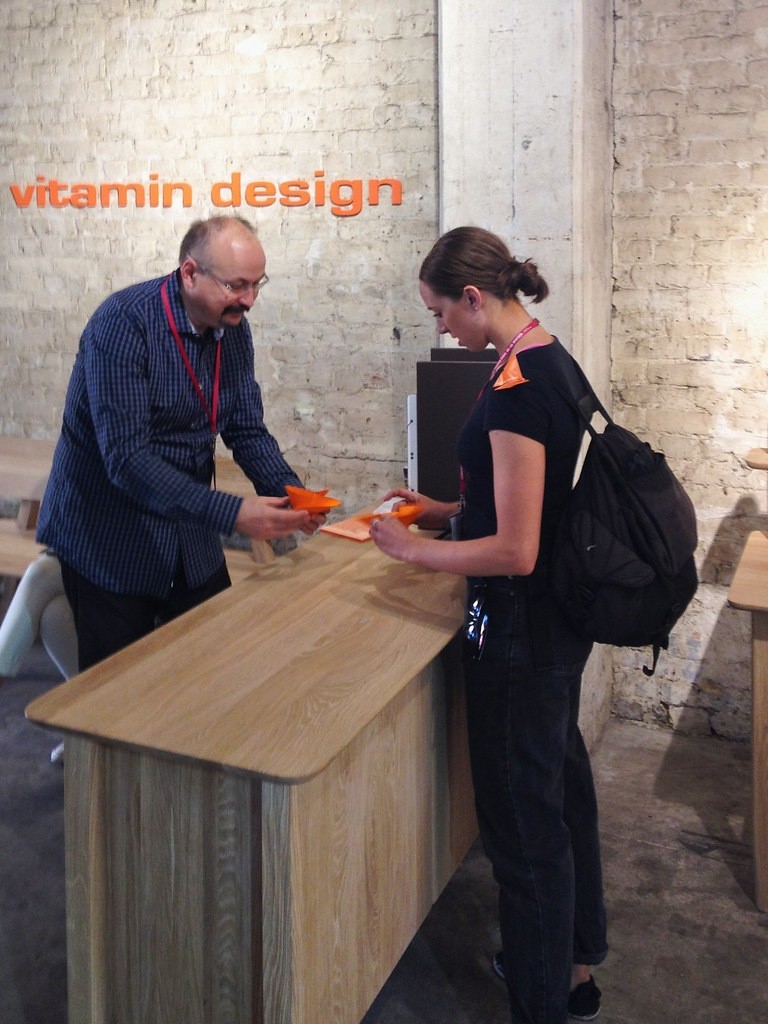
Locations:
[35,213,331,678]
[368,226,612,1024]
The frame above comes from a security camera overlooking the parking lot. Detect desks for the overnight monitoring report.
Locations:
[25,496,480,1024]
[727,531,768,912]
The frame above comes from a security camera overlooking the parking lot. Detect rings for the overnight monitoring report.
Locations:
[372,520,380,526]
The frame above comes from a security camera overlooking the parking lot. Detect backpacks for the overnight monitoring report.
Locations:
[520,359,700,647]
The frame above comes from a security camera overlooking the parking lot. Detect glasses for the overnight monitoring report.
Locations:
[185,253,270,296]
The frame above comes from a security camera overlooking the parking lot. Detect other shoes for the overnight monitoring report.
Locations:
[491,951,602,1022]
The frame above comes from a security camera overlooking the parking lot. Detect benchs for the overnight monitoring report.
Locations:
[0,434,283,588]
